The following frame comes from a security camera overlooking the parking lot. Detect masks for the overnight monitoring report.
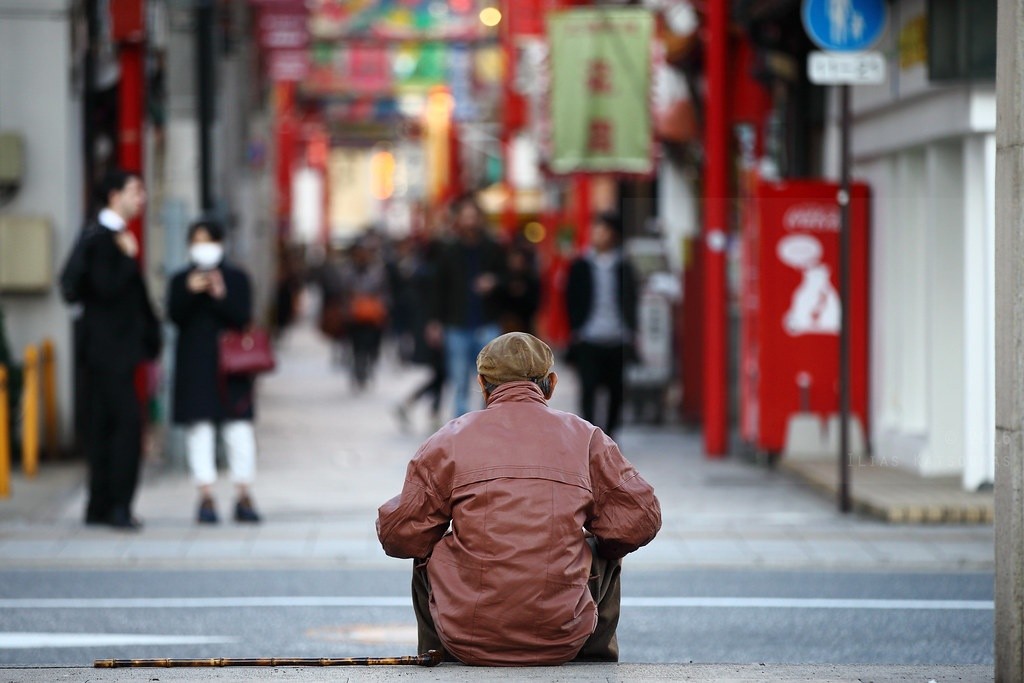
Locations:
[190,242,224,271]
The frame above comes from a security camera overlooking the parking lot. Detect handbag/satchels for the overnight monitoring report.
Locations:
[219,330,275,374]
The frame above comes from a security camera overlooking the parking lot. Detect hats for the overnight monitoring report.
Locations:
[477,331,556,385]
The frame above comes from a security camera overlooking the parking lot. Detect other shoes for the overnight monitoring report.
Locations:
[235,495,259,522]
[200,500,216,522]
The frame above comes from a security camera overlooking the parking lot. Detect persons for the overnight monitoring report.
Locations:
[317,189,648,446]
[375,332,662,667]
[162,218,261,525]
[60,170,163,530]
